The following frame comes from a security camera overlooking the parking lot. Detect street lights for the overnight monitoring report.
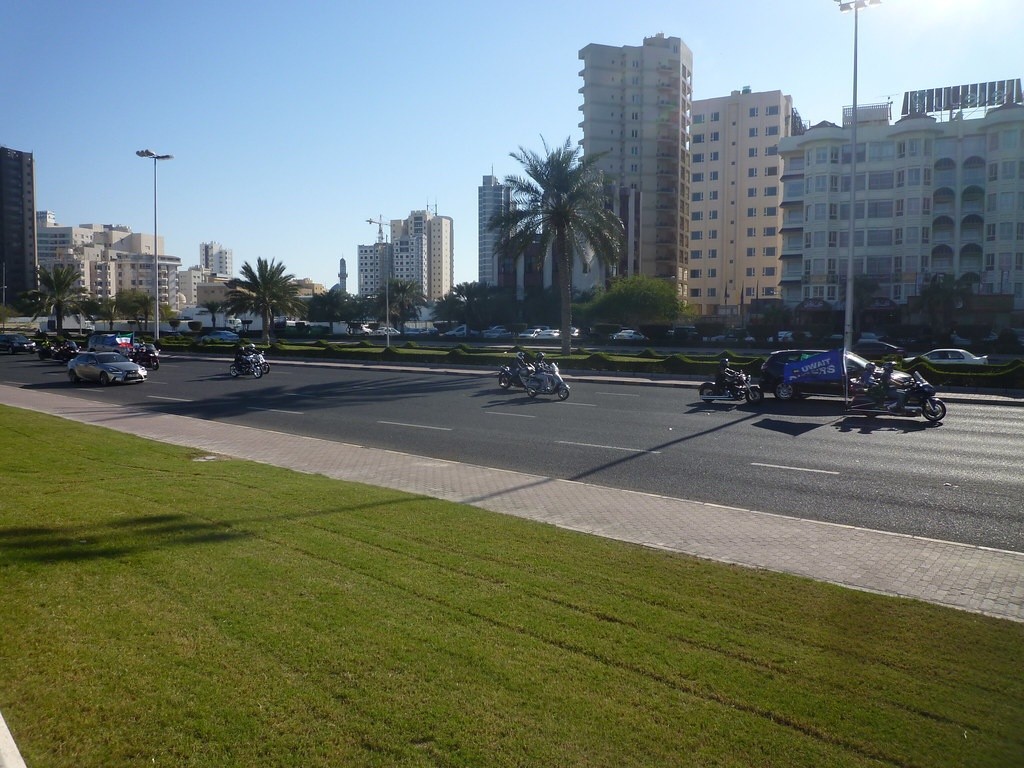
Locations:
[833,0,884,348]
[136,148,174,342]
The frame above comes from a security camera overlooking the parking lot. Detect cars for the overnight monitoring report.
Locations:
[673,326,884,343]
[760,348,913,404]
[351,322,580,340]
[0,333,37,355]
[614,330,650,343]
[903,348,989,366]
[68,350,148,387]
[836,339,908,362]
[201,330,240,345]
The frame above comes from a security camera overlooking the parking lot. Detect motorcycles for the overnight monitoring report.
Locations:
[37,339,162,371]
[698,368,764,406]
[246,351,271,374]
[519,361,570,400]
[496,361,536,391]
[229,352,263,379]
[847,370,947,423]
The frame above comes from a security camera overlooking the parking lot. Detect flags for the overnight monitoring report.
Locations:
[783,349,841,383]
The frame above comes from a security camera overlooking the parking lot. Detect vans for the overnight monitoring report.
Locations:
[88,333,134,359]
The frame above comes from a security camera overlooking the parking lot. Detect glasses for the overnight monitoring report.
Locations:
[866,363,875,372]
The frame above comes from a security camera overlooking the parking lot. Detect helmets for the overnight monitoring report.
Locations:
[536,352,544,360]
[249,343,255,350]
[883,362,897,371]
[720,358,729,368]
[517,352,525,360]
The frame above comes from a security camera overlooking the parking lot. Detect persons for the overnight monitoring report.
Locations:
[715,358,745,400]
[860,362,907,415]
[534,352,553,392]
[42,337,71,361]
[512,352,530,386]
[138,340,148,365]
[236,343,262,375]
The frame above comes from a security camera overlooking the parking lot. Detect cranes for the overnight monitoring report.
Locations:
[367,213,392,278]
